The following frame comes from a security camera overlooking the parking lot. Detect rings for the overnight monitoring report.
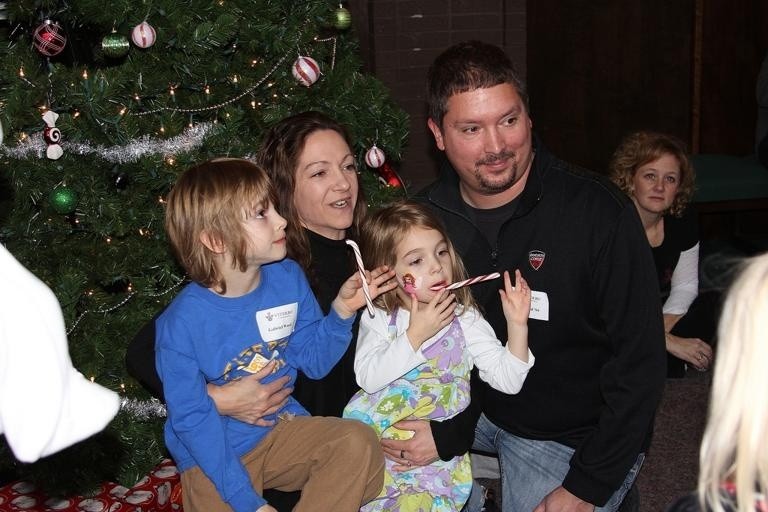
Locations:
[401,452,406,462]
[698,355,703,361]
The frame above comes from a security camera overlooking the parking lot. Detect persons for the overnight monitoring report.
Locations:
[155,154,388,512]
[124,109,482,512]
[342,196,539,511]
[669,250,768,512]
[608,127,713,373]
[408,38,672,511]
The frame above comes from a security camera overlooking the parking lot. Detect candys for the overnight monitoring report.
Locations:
[345,238,376,319]
[429,272,499,292]
